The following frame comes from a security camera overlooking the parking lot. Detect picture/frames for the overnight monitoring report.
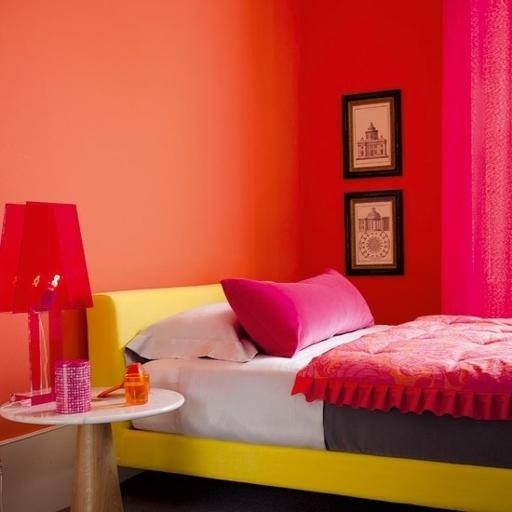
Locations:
[341,89,402,180]
[343,190,404,275]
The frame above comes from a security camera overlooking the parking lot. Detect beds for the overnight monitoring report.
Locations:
[85,284,512,511]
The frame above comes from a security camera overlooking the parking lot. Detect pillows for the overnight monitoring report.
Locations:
[220,268,374,358]
[124,304,258,363]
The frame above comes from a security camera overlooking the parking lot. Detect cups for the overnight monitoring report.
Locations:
[124,373,150,406]
[54,359,92,415]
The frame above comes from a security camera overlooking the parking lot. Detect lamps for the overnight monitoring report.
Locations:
[0,201,95,408]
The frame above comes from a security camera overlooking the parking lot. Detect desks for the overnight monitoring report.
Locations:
[0,388,185,512]
